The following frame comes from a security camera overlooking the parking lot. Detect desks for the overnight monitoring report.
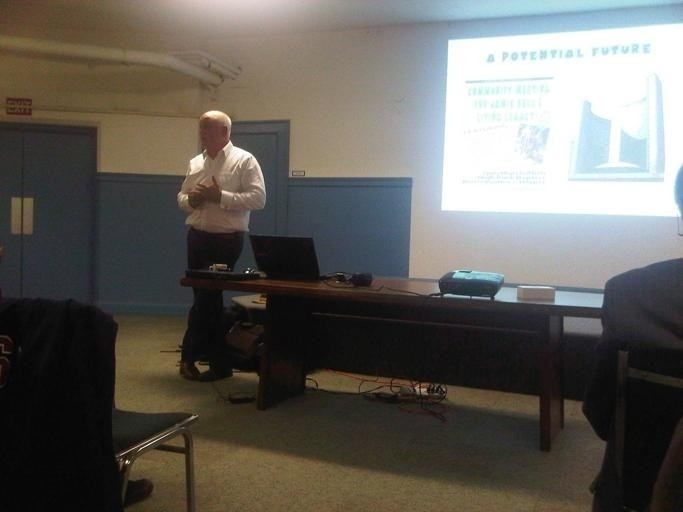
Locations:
[180,260,604,453]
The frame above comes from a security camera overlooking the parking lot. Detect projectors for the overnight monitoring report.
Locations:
[438,268,506,298]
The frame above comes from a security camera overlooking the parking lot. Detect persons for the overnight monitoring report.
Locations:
[176,110,266,382]
[580,163,682,512]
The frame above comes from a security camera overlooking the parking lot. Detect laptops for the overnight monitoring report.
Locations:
[247,232,333,281]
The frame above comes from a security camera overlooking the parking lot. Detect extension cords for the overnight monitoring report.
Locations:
[399,383,446,403]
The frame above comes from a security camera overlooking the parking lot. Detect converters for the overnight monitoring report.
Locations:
[228,391,255,404]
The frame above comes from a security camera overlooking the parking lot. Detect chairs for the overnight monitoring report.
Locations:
[0,298,200,511]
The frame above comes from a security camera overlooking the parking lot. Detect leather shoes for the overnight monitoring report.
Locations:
[180,361,202,381]
[201,368,233,380]
